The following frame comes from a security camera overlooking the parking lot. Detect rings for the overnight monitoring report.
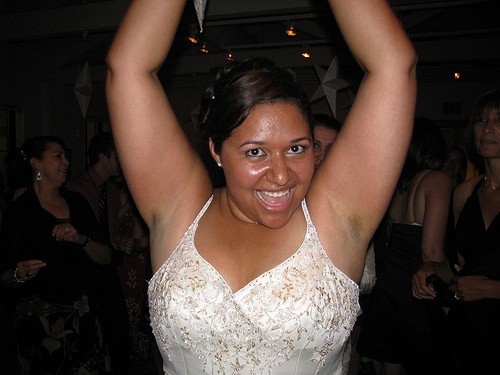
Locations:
[65,227,71,233]
[26,271,31,277]
[454,290,463,300]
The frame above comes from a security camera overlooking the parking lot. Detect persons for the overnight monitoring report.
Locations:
[189,55,342,188]
[105,0,418,375]
[356,88,500,375]
[1,130,165,375]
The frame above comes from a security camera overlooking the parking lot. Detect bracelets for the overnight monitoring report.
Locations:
[81,236,90,247]
[14,267,24,284]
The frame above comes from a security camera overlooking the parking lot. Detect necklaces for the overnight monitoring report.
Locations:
[483,176,500,192]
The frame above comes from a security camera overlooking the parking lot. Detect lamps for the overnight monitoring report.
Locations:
[227,48,232,61]
[301,45,312,60]
[200,38,209,54]
[187,29,199,44]
[286,20,297,37]
